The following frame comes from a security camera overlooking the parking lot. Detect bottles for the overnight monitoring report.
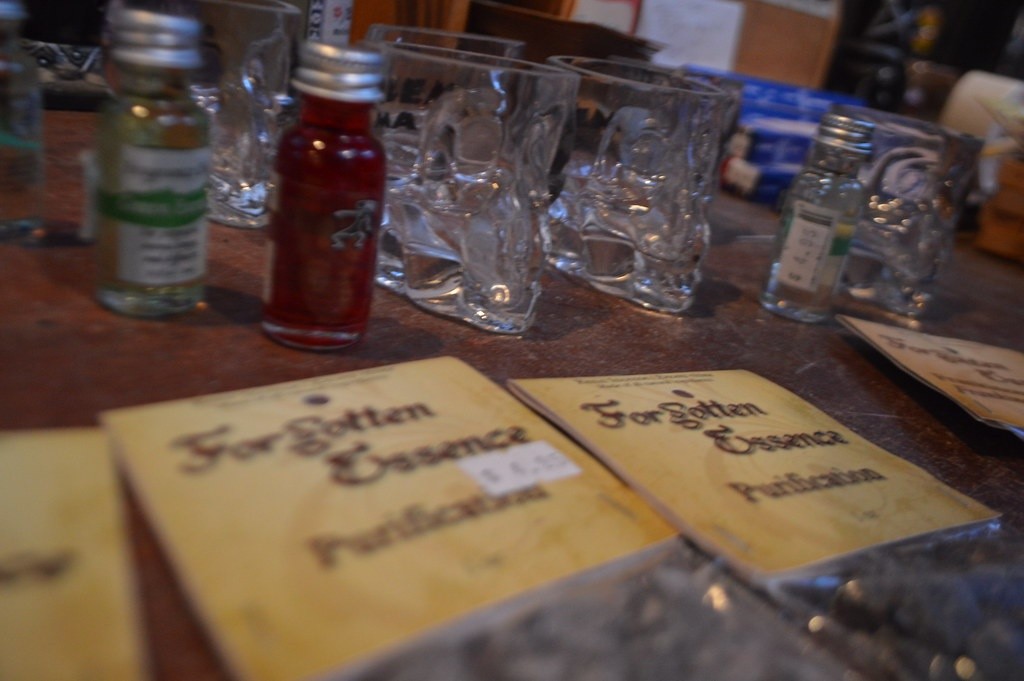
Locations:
[755,114,876,325]
[0,0,47,238]
[94,7,210,318]
[261,39,387,353]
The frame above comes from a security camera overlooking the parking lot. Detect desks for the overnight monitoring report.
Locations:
[0,110,1024,681]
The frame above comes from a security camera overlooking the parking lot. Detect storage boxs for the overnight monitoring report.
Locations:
[352,0,1023,260]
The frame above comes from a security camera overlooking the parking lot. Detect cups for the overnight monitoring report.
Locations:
[370,43,580,333]
[358,25,520,61]
[820,103,985,325]
[542,56,729,314]
[109,0,301,227]
